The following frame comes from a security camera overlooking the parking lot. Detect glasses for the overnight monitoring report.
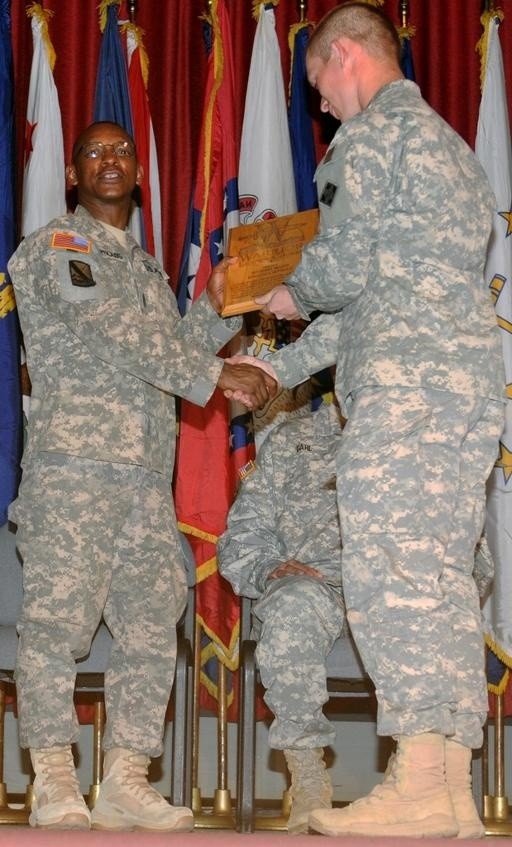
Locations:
[75,140,134,159]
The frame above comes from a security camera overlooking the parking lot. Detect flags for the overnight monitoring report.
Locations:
[0,1,512,696]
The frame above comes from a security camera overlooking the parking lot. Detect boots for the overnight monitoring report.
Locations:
[28,745,91,831]
[90,748,195,833]
[308,731,487,840]
[283,747,332,835]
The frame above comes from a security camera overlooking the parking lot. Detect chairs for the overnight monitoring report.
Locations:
[0,532,194,806]
[235,597,376,835]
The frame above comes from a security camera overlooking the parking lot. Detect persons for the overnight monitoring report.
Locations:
[214,379,495,836]
[223,2,508,842]
[6,120,278,834]
